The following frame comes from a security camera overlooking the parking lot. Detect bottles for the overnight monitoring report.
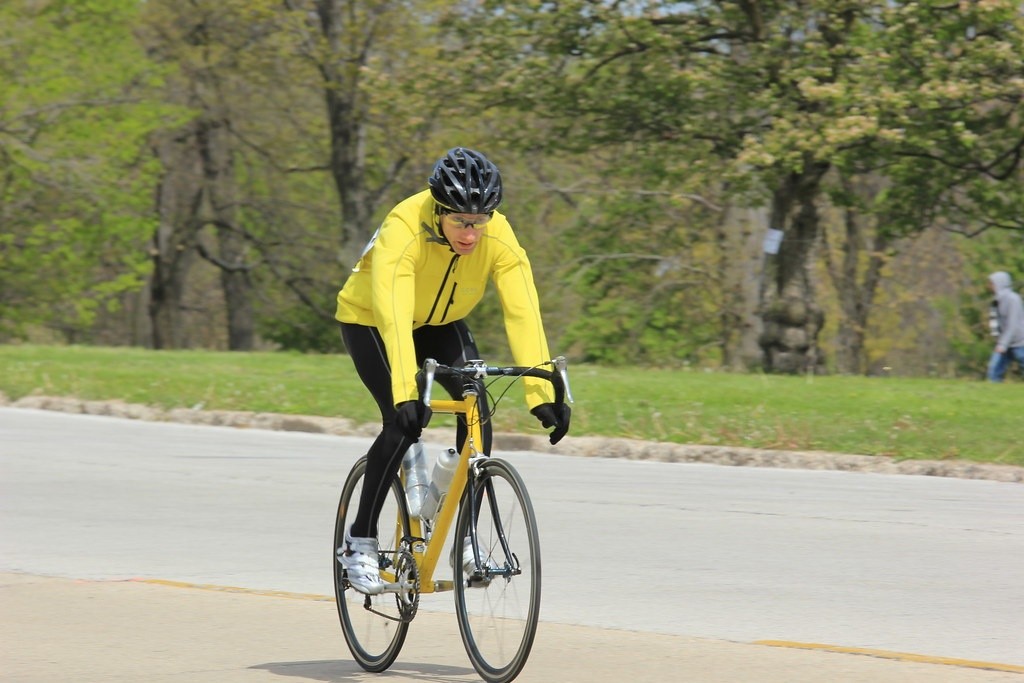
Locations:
[419,448,460,521]
[401,440,430,518]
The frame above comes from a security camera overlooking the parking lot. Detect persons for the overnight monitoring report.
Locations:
[988,272,1024,383]
[336,147,572,595]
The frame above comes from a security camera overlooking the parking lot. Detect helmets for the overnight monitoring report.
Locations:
[427,147,503,214]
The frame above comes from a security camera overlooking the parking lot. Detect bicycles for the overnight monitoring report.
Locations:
[332,354,574,683]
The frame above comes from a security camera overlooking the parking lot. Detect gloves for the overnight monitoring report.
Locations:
[394,400,433,444]
[530,402,572,445]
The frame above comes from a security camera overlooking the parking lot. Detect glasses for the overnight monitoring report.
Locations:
[437,208,496,227]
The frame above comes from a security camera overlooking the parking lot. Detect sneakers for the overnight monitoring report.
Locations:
[336,522,384,596]
[449,535,495,580]
